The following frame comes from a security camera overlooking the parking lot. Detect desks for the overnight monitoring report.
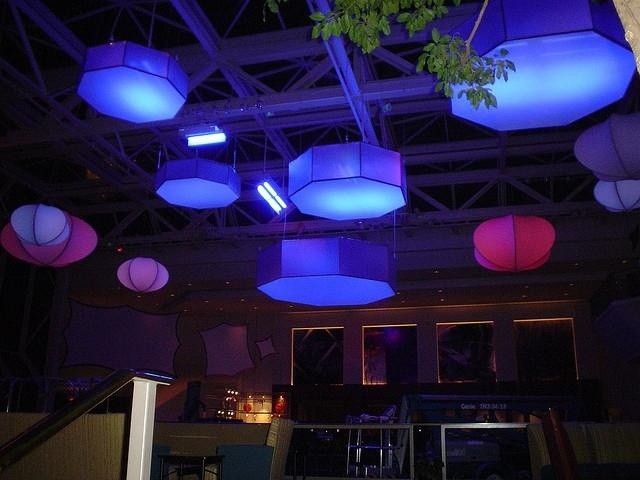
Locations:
[158,453,225,480]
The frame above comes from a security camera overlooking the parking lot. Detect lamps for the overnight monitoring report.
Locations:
[574,114,640,179]
[595,179,640,211]
[74,45,188,126]
[288,143,406,219]
[450,0,637,130]
[257,236,396,305]
[258,182,287,216]
[14,204,67,247]
[474,214,556,271]
[154,159,240,211]
[180,123,225,147]
[7,217,98,268]
[118,258,169,294]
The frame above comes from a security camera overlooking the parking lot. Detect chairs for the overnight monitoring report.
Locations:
[204,418,295,480]
